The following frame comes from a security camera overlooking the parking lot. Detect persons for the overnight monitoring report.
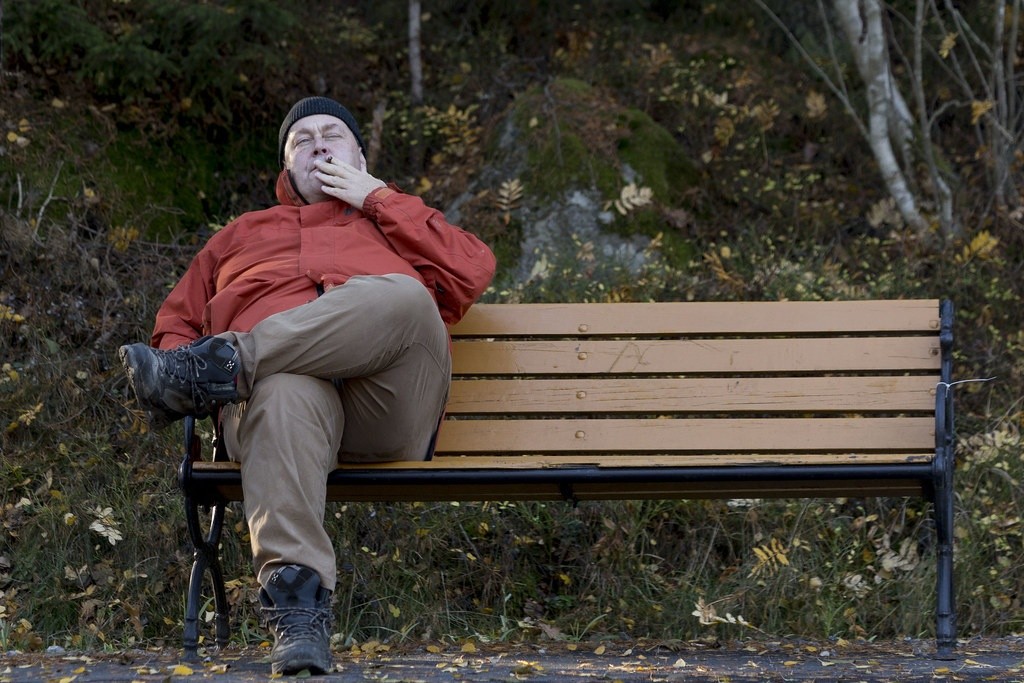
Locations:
[119,95,496,675]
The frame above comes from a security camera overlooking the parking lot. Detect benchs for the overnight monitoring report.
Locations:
[177,298,957,664]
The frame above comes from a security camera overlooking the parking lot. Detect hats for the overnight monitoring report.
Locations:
[279,97,367,171]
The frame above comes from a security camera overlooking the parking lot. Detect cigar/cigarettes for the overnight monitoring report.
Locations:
[326,155,333,164]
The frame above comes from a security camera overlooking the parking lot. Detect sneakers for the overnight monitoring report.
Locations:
[119,334,241,431]
[258,564,333,675]
